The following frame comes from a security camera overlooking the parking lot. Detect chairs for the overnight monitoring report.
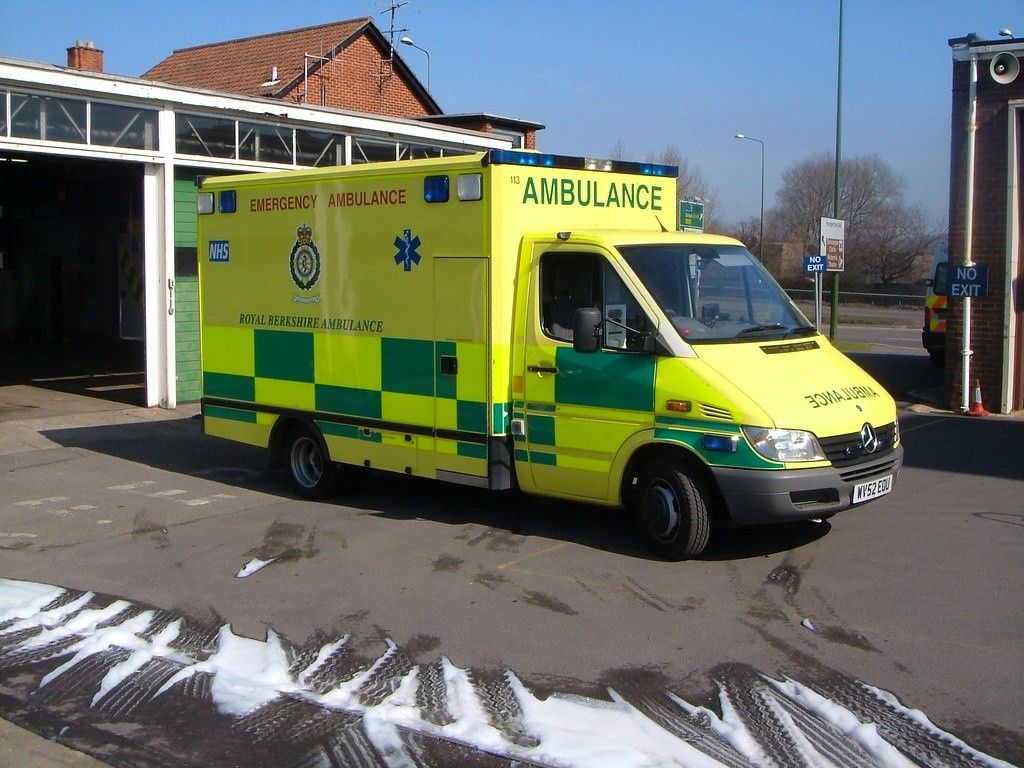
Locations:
[543,258,583,340]
[643,265,680,317]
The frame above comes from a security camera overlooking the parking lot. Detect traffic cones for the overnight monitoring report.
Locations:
[965,377,992,418]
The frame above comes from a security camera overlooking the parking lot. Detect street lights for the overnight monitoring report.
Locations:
[401,35,431,98]
[734,133,766,264]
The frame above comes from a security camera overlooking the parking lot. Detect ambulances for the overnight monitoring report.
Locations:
[192,146,904,564]
[923,240,947,363]
